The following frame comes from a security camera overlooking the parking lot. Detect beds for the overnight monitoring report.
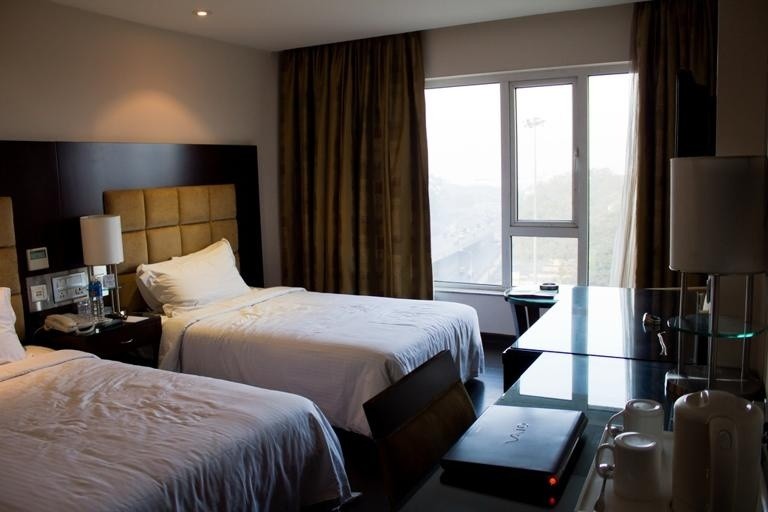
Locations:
[147,282,486,442]
[0,344,357,512]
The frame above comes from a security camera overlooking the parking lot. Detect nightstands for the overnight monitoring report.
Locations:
[35,314,162,369]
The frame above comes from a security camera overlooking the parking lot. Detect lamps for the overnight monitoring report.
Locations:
[665,154,768,399]
[80,212,129,320]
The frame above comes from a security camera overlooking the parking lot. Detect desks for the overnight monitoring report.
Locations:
[499,283,759,398]
[397,353,768,512]
[503,284,563,336]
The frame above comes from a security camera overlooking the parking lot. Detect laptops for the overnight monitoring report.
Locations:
[441,405,588,487]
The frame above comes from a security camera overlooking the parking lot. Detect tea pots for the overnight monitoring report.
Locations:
[673,390,764,511]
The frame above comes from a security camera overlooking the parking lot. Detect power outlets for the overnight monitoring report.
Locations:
[51,272,89,304]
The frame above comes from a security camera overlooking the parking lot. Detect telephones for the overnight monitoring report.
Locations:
[45,313,96,334]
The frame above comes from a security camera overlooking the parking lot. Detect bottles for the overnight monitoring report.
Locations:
[91,278,106,323]
[78,297,92,327]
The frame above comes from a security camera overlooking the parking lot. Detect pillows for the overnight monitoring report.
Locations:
[138,237,254,314]
[0,286,26,367]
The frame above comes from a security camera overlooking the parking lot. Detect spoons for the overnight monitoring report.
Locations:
[593,457,617,511]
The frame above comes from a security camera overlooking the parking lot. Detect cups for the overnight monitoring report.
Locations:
[606,398,665,454]
[595,430,661,501]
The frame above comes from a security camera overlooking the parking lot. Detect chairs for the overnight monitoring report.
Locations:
[361,349,478,512]
